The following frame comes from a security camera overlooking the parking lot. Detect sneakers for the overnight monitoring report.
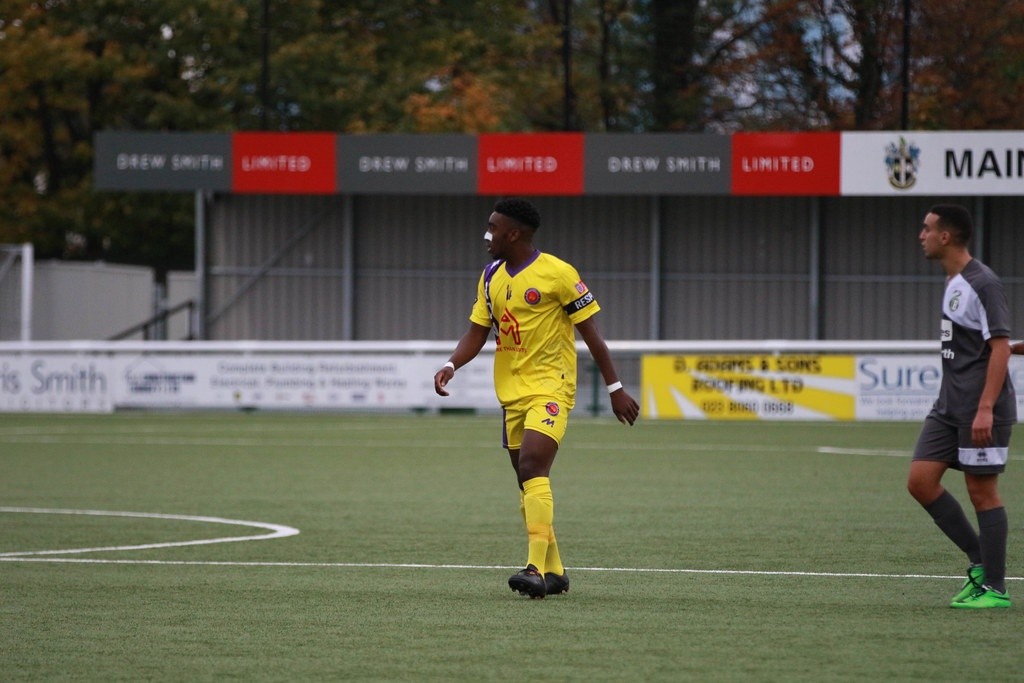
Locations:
[952,567,985,601]
[544,569,570,595]
[949,584,1011,607]
[508,564,546,599]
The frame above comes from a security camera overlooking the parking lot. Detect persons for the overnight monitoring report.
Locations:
[432,199,639,597]
[905,205,1018,608]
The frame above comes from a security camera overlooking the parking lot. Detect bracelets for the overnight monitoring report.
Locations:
[606,381,623,393]
[443,361,455,370]
